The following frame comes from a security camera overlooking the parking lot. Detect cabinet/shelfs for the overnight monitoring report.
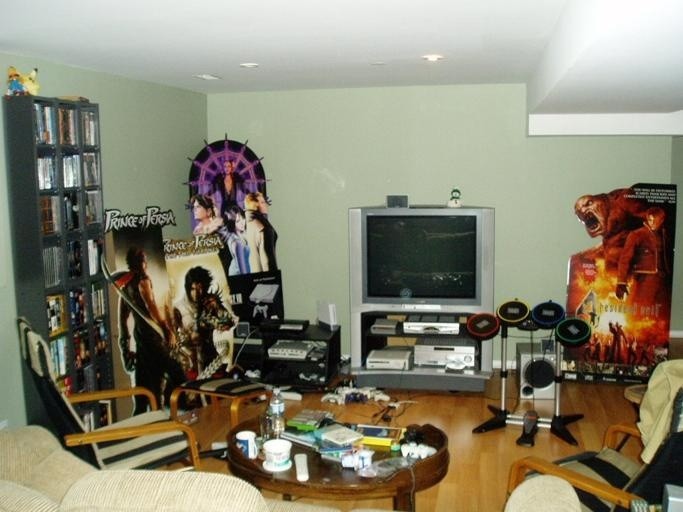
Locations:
[349,302,497,395]
[0,93,119,450]
[215,322,340,389]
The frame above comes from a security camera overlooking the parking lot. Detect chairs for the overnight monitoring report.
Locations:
[503,356,683,512]
[13,314,200,477]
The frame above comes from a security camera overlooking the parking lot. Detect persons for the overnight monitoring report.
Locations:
[190,161,278,277]
[174,266,234,381]
[615,207,669,318]
[584,321,651,367]
[118,247,185,416]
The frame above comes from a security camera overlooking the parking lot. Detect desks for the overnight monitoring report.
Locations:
[225,414,451,510]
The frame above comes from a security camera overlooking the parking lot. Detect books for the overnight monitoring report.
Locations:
[34,102,112,433]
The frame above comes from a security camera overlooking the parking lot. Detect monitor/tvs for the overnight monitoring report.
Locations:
[355,210,485,305]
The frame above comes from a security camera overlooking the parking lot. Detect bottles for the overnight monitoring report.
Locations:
[260,387,286,439]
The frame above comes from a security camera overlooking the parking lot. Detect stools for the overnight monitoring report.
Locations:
[169,376,275,432]
[614,379,648,452]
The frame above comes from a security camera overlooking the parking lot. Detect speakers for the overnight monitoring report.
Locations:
[516,342,557,400]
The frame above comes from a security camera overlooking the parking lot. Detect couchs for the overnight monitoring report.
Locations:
[0,422,350,511]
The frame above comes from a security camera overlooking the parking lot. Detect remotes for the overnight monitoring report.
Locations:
[293,453,310,482]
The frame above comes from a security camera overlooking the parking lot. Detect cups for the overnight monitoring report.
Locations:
[235,429,259,462]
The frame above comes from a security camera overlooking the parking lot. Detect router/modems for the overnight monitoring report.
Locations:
[316,300,338,332]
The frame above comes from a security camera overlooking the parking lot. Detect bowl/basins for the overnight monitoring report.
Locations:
[261,439,292,467]
